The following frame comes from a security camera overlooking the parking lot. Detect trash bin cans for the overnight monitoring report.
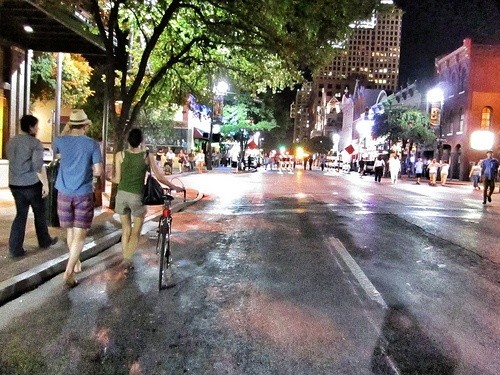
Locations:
[38,162,66,228]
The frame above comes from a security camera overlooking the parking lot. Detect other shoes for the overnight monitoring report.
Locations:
[62,263,83,288]
[40,236,59,248]
[118,259,135,278]
[358,176,492,205]
[8,248,27,259]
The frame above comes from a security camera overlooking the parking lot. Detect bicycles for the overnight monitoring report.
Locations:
[153,186,187,291]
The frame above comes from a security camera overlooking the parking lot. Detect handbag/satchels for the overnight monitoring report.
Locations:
[141,175,165,205]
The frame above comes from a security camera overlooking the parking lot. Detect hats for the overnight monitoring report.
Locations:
[66,108,91,126]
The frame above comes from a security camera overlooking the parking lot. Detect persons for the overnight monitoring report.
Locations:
[52,109,104,287]
[154,147,500,205]
[6,115,58,258]
[105,129,184,274]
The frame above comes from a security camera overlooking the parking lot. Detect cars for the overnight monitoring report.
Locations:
[325,156,343,170]
[342,162,359,170]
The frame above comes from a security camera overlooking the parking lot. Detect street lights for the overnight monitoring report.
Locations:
[470,128,495,152]
[428,85,444,180]
[206,80,232,170]
